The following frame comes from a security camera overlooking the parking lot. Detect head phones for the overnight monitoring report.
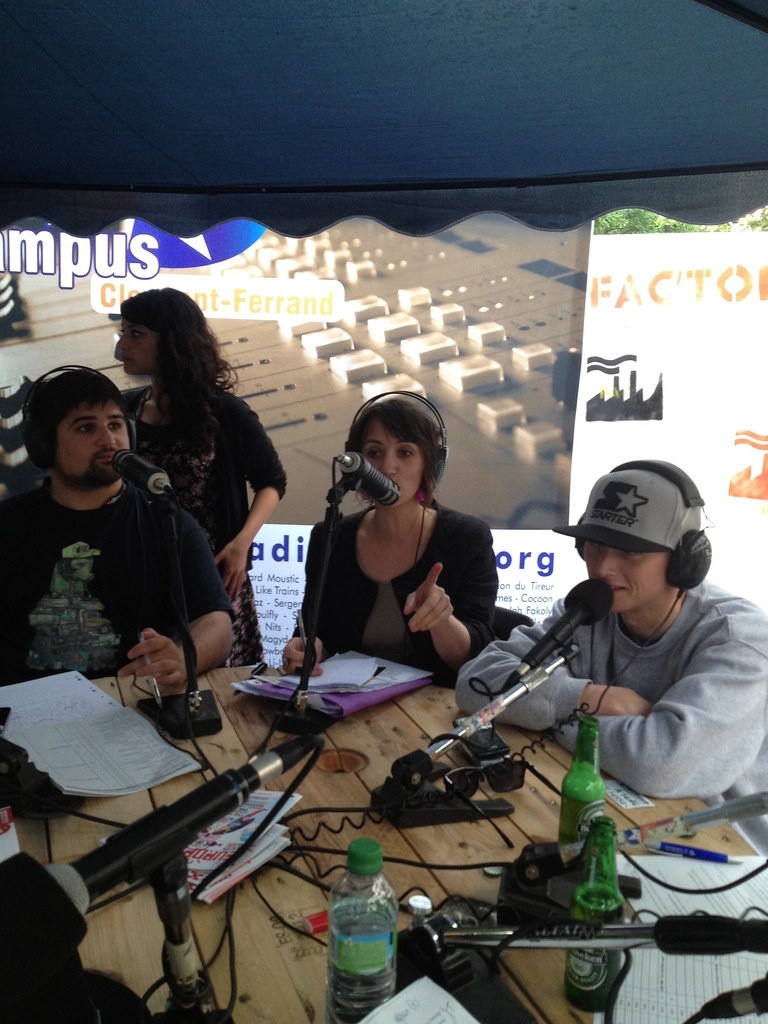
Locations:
[343,391,449,482]
[576,461,712,590]
[21,365,137,469]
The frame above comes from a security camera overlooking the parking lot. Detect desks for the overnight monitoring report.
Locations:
[0,663,768,1024]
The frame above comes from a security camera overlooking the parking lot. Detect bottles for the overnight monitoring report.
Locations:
[562,816,621,1012]
[323,837,396,1024]
[558,716,606,855]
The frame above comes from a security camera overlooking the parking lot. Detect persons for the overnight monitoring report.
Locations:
[283,398,499,691]
[118,288,287,670]
[456,460,768,861]
[0,371,233,687]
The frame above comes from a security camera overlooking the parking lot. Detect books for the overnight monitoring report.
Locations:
[164,791,304,903]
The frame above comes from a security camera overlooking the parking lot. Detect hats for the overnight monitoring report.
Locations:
[551,460,702,556]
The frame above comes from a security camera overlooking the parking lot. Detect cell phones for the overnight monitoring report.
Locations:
[453,717,510,759]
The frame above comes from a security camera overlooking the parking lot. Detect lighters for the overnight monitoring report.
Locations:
[306,912,329,934]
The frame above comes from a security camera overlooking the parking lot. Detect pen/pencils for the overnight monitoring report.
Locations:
[644,839,746,864]
[232,662,268,695]
[297,608,306,651]
[139,633,162,707]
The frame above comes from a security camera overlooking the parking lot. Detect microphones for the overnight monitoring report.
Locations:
[700,974,768,1022]
[500,579,614,692]
[0,734,320,974]
[111,449,174,497]
[336,451,400,506]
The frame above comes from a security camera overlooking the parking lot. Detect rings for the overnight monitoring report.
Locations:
[282,656,290,666]
